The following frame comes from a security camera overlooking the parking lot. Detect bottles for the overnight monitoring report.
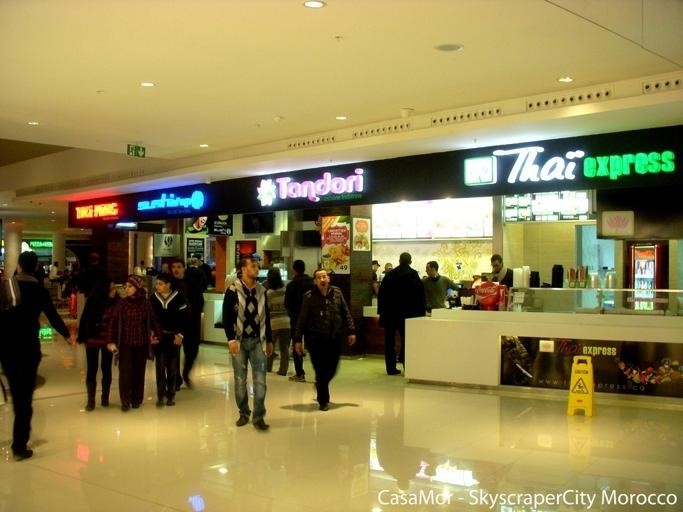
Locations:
[600,266,608,289]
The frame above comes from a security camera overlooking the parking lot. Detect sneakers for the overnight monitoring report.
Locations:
[320,403,329,411]
[13,450,33,460]
[254,419,269,430]
[237,415,248,426]
[122,400,139,411]
[387,369,401,375]
[157,376,190,405]
[289,375,305,382]
[277,372,286,376]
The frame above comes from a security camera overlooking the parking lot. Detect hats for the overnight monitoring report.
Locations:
[372,261,380,267]
[128,275,144,289]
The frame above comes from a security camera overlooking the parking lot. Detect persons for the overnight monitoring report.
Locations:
[284,259,315,382]
[0,251,212,458]
[294,268,358,411]
[377,251,427,375]
[420,260,460,308]
[372,260,381,295]
[185,216,209,234]
[266,266,292,376]
[379,262,394,281]
[221,256,274,431]
[488,254,513,288]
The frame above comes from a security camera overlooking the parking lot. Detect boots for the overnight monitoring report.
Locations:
[85,383,96,410]
[102,378,111,406]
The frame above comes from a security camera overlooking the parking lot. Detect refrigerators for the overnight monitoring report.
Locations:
[627,243,663,311]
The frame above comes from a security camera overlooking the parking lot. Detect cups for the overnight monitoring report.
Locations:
[322,256,332,272]
[608,272,616,289]
[590,274,598,289]
